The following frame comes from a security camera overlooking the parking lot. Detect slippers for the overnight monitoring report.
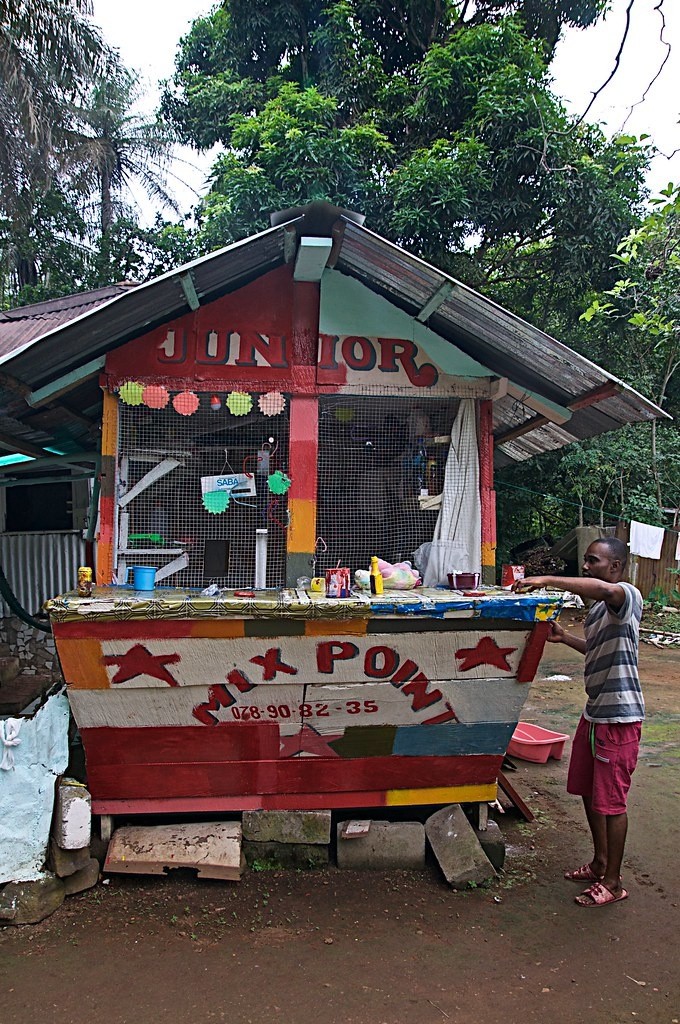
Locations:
[575,881,629,907]
[563,863,623,883]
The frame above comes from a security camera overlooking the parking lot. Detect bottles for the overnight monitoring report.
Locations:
[370,556,384,595]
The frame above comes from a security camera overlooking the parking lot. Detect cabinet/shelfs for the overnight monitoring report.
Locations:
[41,585,563,843]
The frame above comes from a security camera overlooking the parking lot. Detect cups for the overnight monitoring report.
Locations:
[133,566,156,590]
[79,566,92,597]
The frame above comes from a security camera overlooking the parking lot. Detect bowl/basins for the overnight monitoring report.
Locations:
[447,572,480,588]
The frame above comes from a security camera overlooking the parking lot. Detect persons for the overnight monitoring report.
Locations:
[509,538,645,908]
